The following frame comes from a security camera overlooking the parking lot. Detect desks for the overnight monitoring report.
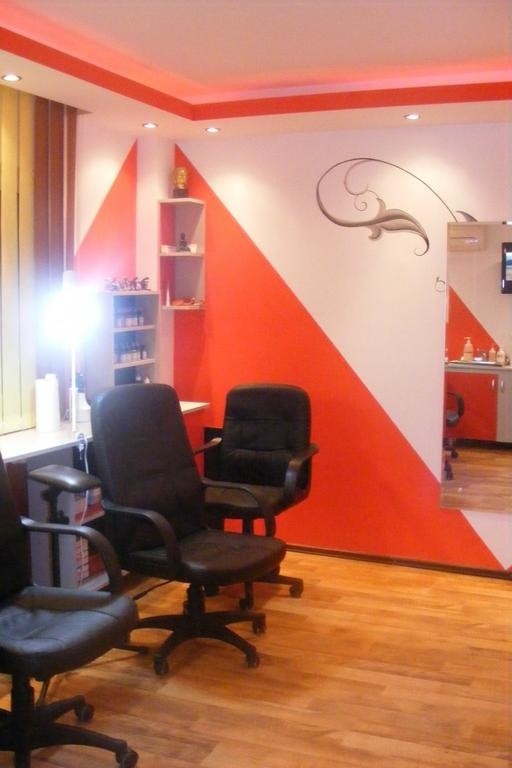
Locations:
[0,401,206,593]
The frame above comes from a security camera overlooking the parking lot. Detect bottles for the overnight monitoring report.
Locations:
[120,342,147,362]
[116,306,144,328]
[135,373,142,384]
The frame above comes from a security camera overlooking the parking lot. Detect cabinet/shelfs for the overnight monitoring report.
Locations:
[158,198,205,311]
[97,288,160,392]
[445,358,512,449]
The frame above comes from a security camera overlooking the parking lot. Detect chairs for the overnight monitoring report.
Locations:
[202,386,320,608]
[94,382,287,676]
[441,387,464,479]
[0,455,140,768]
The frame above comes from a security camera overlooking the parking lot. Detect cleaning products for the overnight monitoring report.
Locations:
[463,337,473,361]
